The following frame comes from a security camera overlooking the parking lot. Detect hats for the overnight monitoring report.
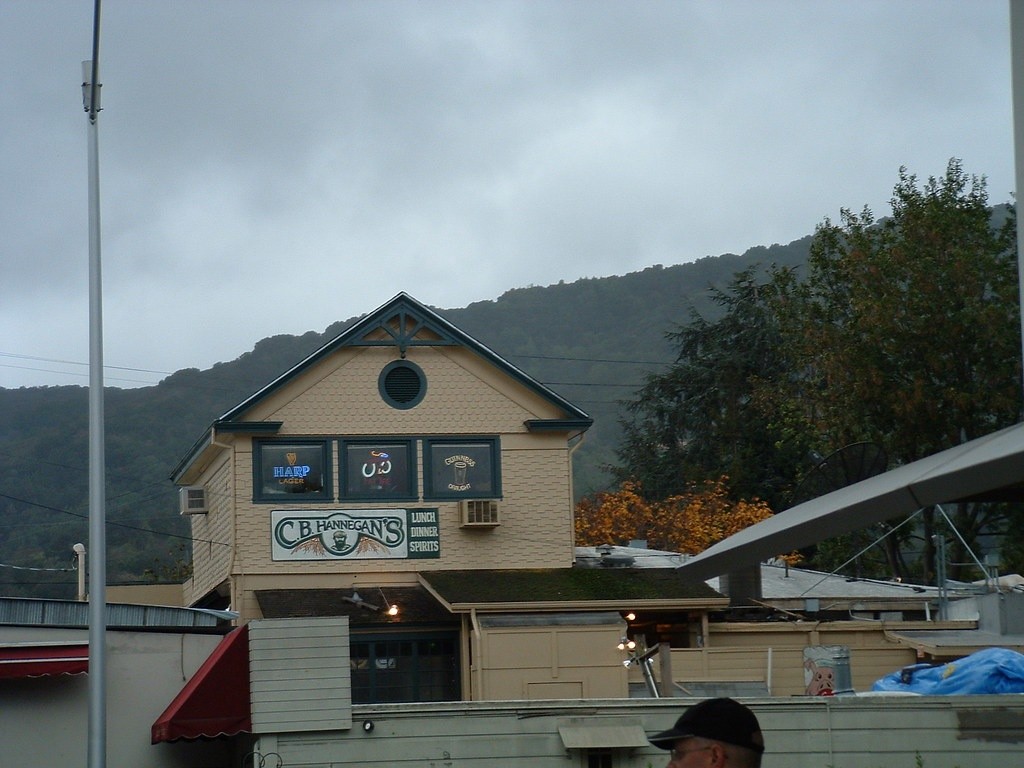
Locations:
[647,698,765,754]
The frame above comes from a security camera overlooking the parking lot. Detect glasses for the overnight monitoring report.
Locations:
[670,746,729,762]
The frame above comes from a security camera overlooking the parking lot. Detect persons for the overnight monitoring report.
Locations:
[647,697,765,768]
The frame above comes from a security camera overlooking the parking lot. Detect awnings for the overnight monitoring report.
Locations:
[0,645,89,686]
[150,623,249,746]
